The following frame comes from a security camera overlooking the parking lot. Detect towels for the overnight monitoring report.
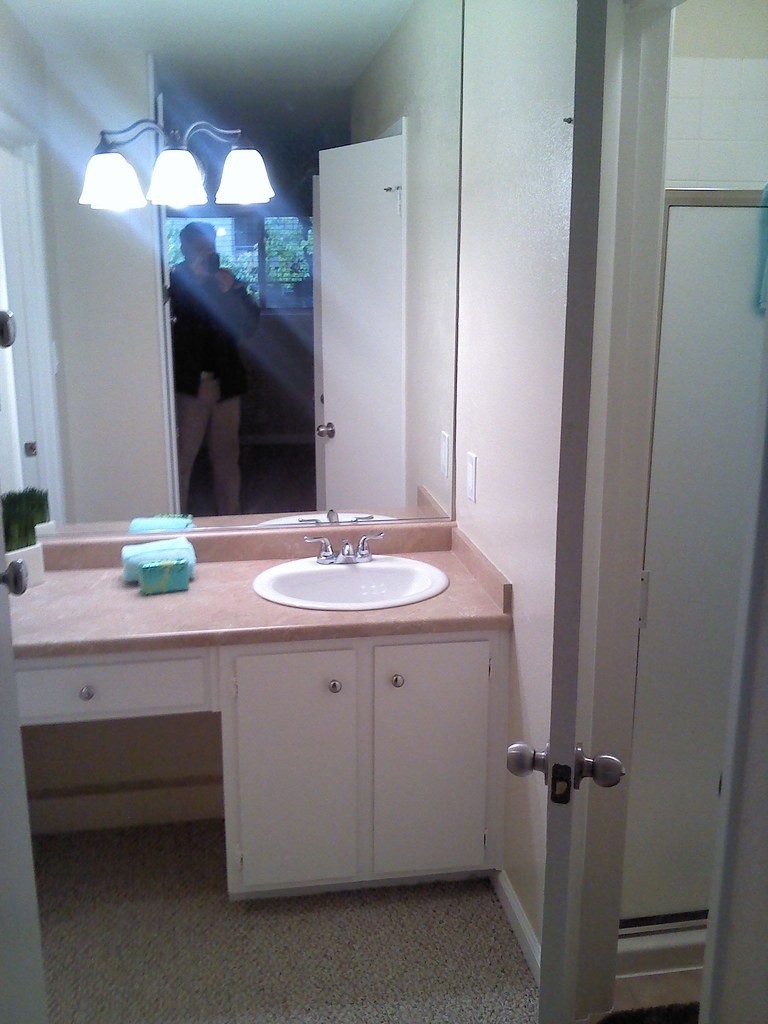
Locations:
[750,181,768,321]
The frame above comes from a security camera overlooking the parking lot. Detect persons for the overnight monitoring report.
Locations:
[169,222,261,515]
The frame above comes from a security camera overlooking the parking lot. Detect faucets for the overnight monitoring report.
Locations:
[334,541,356,563]
[327,509,340,522]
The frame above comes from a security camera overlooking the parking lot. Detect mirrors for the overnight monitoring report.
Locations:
[0,0,465,543]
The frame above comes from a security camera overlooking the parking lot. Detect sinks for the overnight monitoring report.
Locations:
[254,512,400,525]
[251,554,450,611]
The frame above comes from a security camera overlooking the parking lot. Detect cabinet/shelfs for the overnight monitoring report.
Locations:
[220,630,508,901]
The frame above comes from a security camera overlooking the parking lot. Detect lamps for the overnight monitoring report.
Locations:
[77,119,275,211]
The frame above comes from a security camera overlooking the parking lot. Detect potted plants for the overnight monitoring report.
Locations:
[23,485,57,537]
[0,490,45,590]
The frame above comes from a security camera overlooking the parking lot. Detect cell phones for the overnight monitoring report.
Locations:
[207,253,220,274]
